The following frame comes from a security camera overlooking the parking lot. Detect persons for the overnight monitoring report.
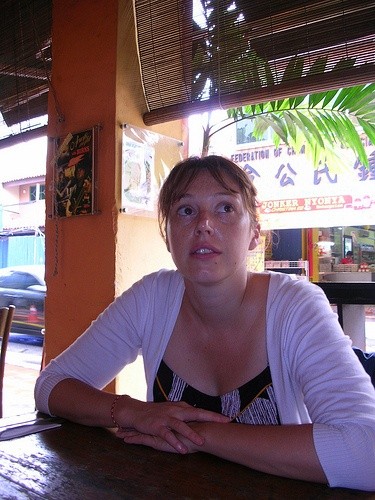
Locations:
[341,251,355,264]
[34,156,374,491]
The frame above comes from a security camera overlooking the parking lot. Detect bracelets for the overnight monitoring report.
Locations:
[111,395,131,428]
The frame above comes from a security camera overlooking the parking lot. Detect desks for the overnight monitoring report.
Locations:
[0,416,375,500]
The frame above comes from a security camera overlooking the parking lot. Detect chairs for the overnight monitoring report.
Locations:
[0,305,16,418]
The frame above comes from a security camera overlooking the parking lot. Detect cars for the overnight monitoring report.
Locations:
[0,268,47,336]
